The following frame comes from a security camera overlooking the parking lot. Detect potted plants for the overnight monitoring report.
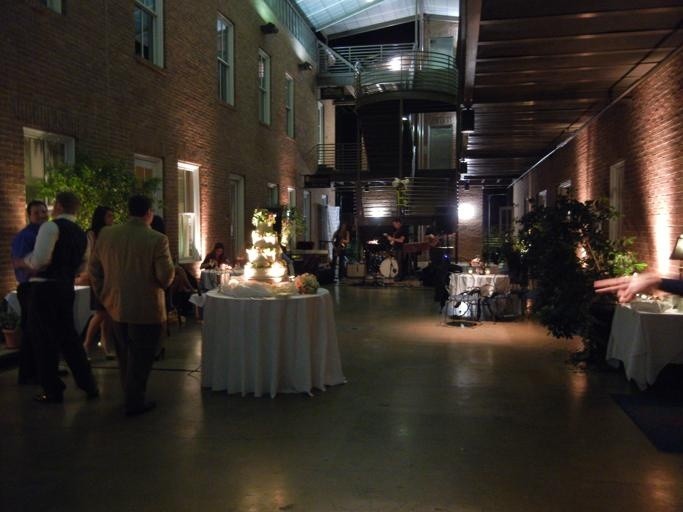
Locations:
[0,307,21,349]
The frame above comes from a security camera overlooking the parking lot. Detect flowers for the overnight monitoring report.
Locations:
[295,272,320,295]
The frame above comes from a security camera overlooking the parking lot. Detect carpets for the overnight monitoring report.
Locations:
[609,392,683,452]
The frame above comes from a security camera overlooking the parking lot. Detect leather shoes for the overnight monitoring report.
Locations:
[86,385,157,418]
[31,390,64,404]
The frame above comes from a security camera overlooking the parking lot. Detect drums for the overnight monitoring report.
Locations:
[375,255,399,278]
[346,262,367,278]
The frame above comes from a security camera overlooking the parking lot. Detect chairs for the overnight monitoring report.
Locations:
[441,271,527,328]
[169,265,201,320]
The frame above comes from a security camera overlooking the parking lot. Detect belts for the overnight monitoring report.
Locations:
[27,276,55,283]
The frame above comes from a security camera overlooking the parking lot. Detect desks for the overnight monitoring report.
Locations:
[612,294,683,395]
[7,285,92,339]
[204,287,330,397]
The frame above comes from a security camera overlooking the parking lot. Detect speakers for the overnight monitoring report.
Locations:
[435,205,456,236]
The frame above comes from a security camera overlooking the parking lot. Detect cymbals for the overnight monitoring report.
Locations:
[367,240,380,245]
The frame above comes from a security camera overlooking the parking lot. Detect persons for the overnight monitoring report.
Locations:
[303,216,458,287]
[617,270,681,305]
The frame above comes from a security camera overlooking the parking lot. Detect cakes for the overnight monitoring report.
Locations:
[244,210,288,278]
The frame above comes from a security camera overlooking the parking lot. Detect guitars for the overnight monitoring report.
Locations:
[382,232,397,248]
[334,238,345,248]
